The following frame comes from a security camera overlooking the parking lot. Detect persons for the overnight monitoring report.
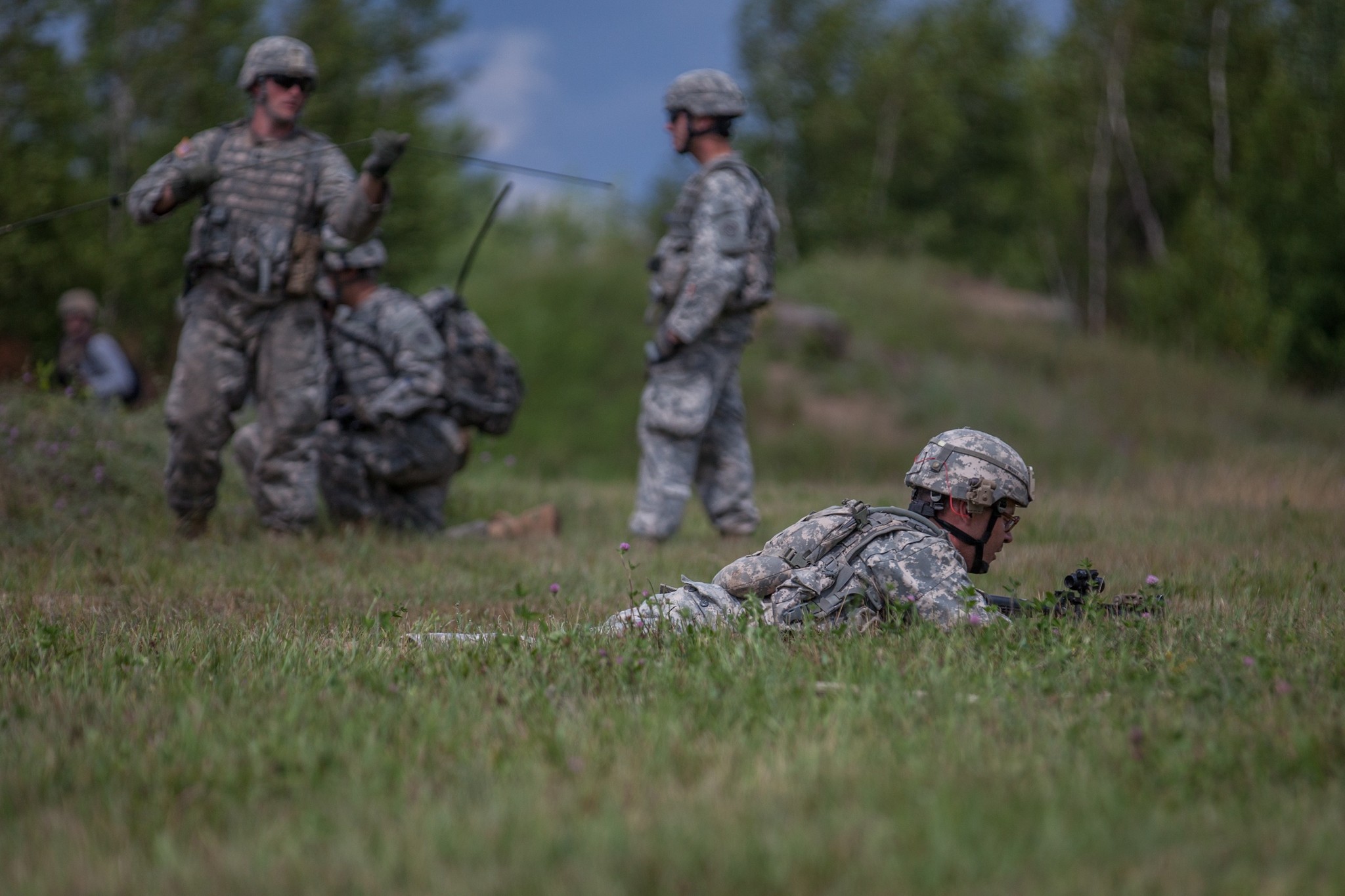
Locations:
[60,35,565,544]
[402,430,1039,645]
[628,69,776,543]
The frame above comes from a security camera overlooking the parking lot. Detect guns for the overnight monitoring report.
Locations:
[979,560,1170,615]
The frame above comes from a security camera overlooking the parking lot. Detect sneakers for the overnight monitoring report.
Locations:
[496,503,561,543]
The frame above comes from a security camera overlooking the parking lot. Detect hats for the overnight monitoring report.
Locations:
[57,289,102,321]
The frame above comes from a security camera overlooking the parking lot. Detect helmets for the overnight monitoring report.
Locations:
[904,429,1033,507]
[238,36,324,92]
[665,68,749,117]
[323,237,386,266]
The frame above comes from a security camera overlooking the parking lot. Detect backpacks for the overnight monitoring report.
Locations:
[419,283,525,434]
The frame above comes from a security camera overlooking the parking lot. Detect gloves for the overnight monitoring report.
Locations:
[171,164,220,205]
[642,330,676,364]
[364,129,410,178]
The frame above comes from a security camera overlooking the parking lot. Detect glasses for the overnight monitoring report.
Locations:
[272,73,317,98]
[667,113,678,122]
[1002,513,1021,531]
